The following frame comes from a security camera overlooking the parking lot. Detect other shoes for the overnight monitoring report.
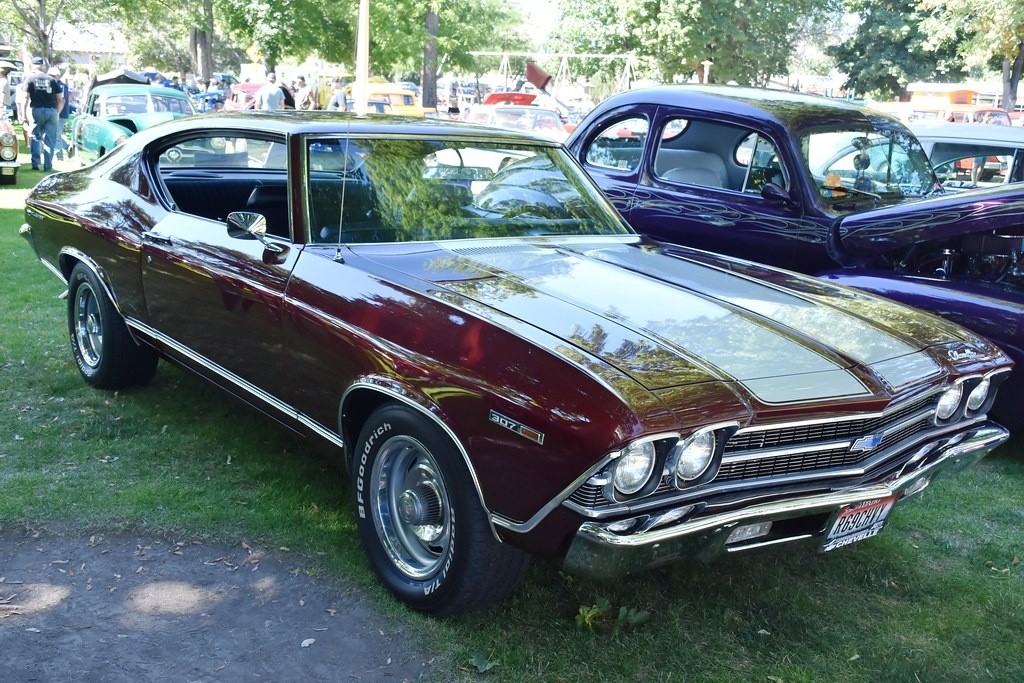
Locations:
[68,147,72,158]
[32,166,39,169]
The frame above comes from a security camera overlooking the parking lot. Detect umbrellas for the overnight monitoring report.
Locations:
[0,61,18,69]
[86,68,152,88]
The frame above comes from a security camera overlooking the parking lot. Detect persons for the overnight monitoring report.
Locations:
[327,79,348,112]
[152,73,231,99]
[946,112,955,124]
[447,88,460,117]
[14,57,75,172]
[295,75,316,111]
[973,156,987,187]
[0,68,12,108]
[255,72,286,112]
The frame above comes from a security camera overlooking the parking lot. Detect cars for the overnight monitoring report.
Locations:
[71,83,228,169]
[17,109,1016,621]
[0,47,27,185]
[816,101,1024,197]
[193,73,690,143]
[477,84,1024,444]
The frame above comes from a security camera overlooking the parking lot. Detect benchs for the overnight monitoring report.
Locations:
[163,176,387,244]
[590,147,730,190]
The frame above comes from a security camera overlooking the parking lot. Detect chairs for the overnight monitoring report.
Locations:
[247,184,325,240]
[395,182,475,219]
[662,167,723,189]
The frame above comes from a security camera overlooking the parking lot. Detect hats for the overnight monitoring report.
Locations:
[32,57,44,65]
[267,73,277,82]
[47,68,61,76]
